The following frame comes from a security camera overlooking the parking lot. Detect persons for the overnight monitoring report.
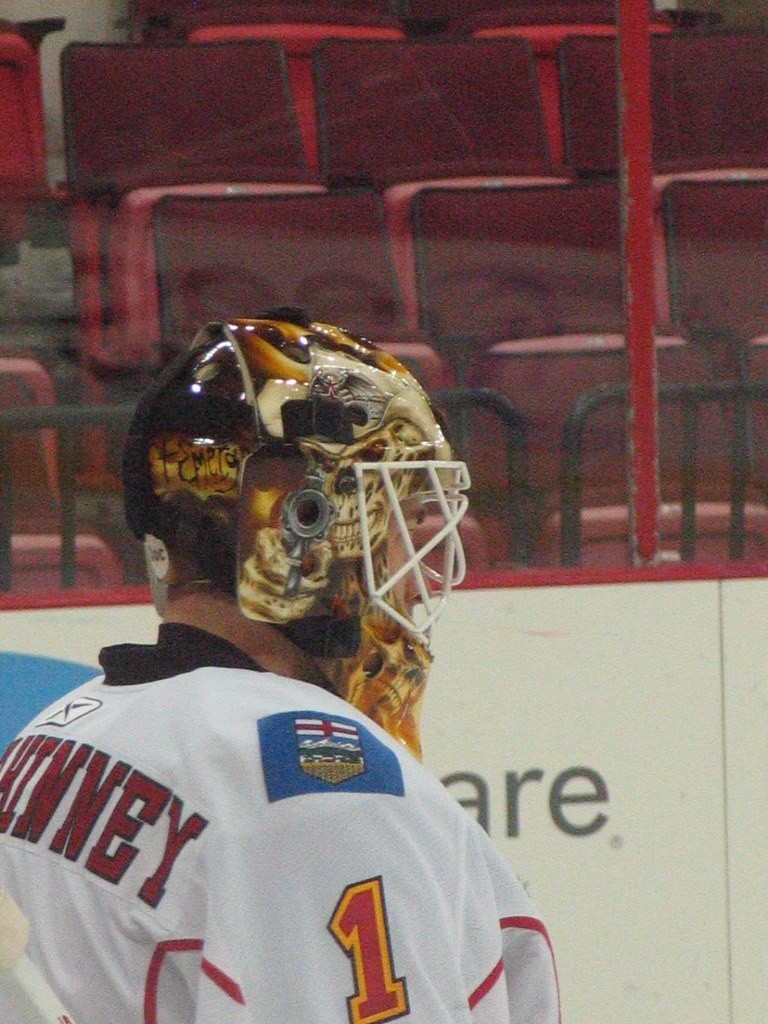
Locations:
[1,305,564,1023]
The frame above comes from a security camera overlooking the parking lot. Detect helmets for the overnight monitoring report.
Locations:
[126,304,470,764]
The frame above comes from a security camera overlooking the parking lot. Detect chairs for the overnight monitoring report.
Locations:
[0,0,768,592]
